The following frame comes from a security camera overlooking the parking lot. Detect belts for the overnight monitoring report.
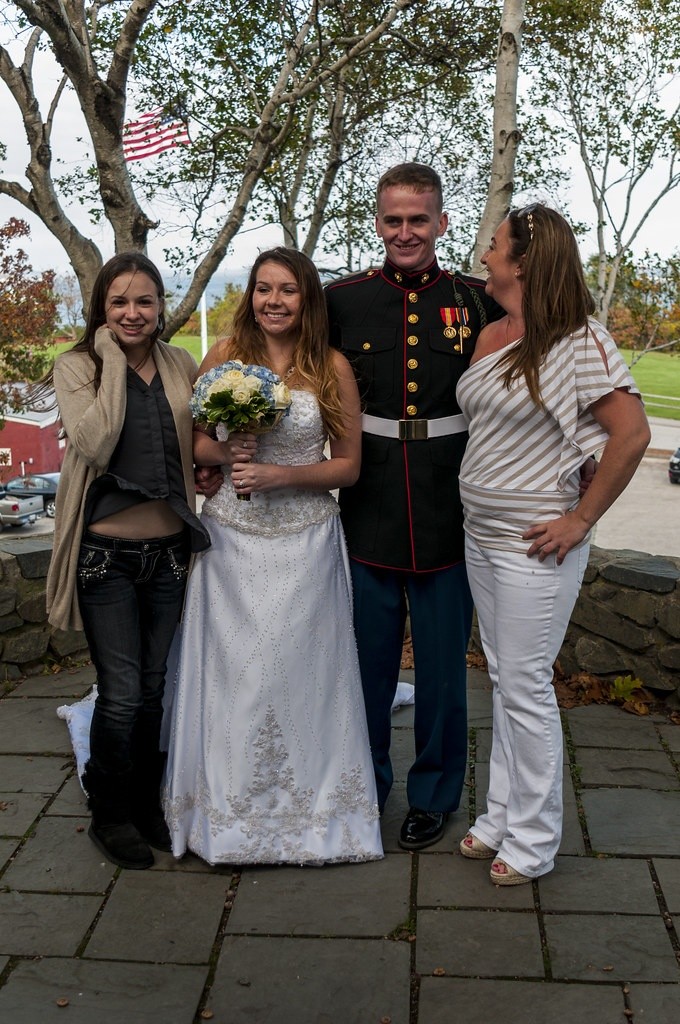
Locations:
[361,413,468,441]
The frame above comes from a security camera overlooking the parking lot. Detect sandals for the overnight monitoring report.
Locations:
[460,830,498,858]
[490,857,532,884]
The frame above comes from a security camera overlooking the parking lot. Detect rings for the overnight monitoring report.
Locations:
[243,441,248,448]
[238,479,244,488]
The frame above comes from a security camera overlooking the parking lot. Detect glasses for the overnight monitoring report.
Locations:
[517,202,538,240]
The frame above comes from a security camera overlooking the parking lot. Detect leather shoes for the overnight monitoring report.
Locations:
[397,808,448,850]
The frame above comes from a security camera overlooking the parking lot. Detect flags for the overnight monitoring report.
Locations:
[119,92,192,162]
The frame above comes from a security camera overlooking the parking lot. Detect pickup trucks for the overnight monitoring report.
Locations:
[0,481,45,534]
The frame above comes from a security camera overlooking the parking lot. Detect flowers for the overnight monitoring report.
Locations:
[188,360,293,503]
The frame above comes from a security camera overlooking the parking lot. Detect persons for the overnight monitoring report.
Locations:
[47,252,200,870]
[454,205,652,885]
[196,162,599,851]
[194,248,362,860]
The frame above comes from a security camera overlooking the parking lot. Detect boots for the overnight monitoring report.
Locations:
[123,768,171,852]
[80,774,155,869]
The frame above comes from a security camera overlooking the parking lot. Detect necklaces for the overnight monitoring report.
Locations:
[505,315,526,346]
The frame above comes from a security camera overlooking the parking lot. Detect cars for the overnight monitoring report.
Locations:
[668,447,680,485]
[0,472,62,518]
